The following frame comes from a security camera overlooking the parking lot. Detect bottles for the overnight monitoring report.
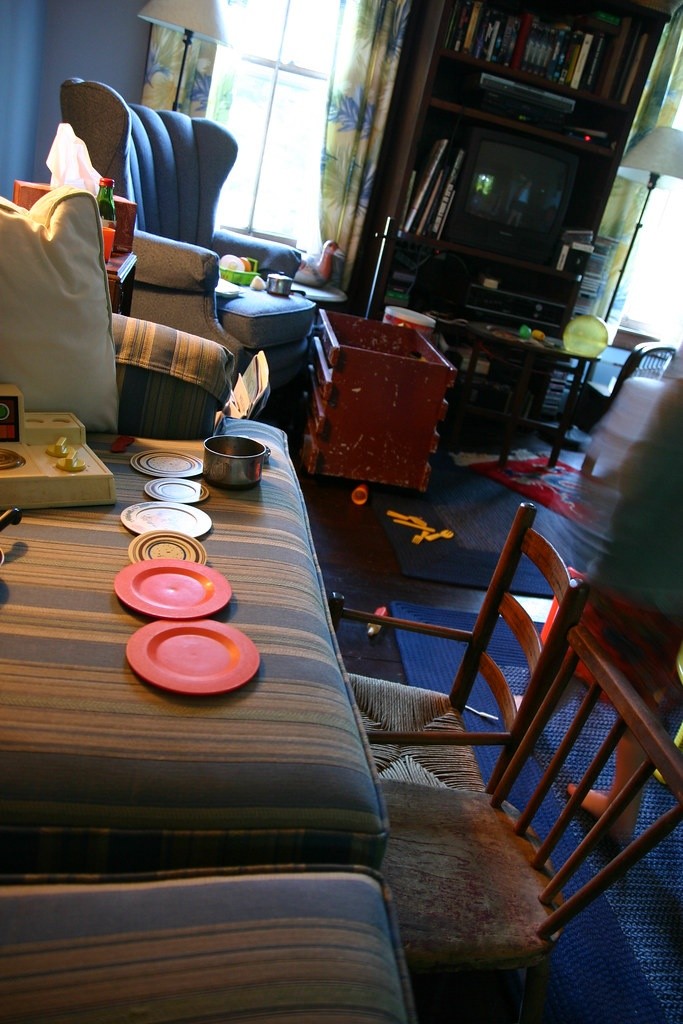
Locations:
[96,177,117,230]
[367,607,389,637]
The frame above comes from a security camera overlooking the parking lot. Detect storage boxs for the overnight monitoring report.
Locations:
[556,241,595,274]
[299,309,457,492]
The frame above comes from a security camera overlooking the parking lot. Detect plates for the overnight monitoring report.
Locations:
[120,501,212,539]
[125,619,260,695]
[114,559,232,619]
[220,254,245,272]
[144,478,210,504]
[130,449,203,478]
[127,530,208,566]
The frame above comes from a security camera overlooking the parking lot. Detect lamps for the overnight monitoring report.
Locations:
[603,125,683,323]
[137,0,228,111]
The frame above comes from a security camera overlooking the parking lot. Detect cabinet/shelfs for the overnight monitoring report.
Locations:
[348,0,673,431]
[543,235,620,414]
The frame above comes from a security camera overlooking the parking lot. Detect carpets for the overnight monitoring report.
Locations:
[370,433,622,598]
[450,449,623,543]
[392,600,683,1023]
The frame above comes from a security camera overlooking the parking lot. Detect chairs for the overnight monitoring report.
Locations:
[328,502,590,795]
[379,625,683,1023]
[576,341,678,474]
[61,78,315,393]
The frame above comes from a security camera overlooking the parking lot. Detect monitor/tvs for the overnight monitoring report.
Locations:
[442,126,580,266]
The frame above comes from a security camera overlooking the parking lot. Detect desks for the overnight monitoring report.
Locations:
[452,322,600,469]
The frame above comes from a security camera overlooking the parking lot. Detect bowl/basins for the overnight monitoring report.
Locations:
[266,273,292,296]
[203,434,266,490]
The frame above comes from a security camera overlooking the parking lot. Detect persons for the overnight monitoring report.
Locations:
[568,350,683,840]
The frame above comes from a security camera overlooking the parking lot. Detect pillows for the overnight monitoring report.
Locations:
[0,185,119,436]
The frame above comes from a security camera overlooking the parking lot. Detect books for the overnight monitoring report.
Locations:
[399,0,614,240]
[215,350,270,436]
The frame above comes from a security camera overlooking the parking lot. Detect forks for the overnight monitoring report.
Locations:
[412,530,429,545]
[425,529,453,542]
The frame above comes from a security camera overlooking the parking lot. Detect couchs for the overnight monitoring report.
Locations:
[0,313,419,1024]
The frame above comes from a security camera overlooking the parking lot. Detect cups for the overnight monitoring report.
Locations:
[101,226,116,264]
[352,484,368,505]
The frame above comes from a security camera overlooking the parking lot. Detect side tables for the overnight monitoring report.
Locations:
[106,251,137,317]
[291,281,348,302]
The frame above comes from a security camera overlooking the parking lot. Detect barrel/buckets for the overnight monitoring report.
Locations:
[382,306,436,342]
